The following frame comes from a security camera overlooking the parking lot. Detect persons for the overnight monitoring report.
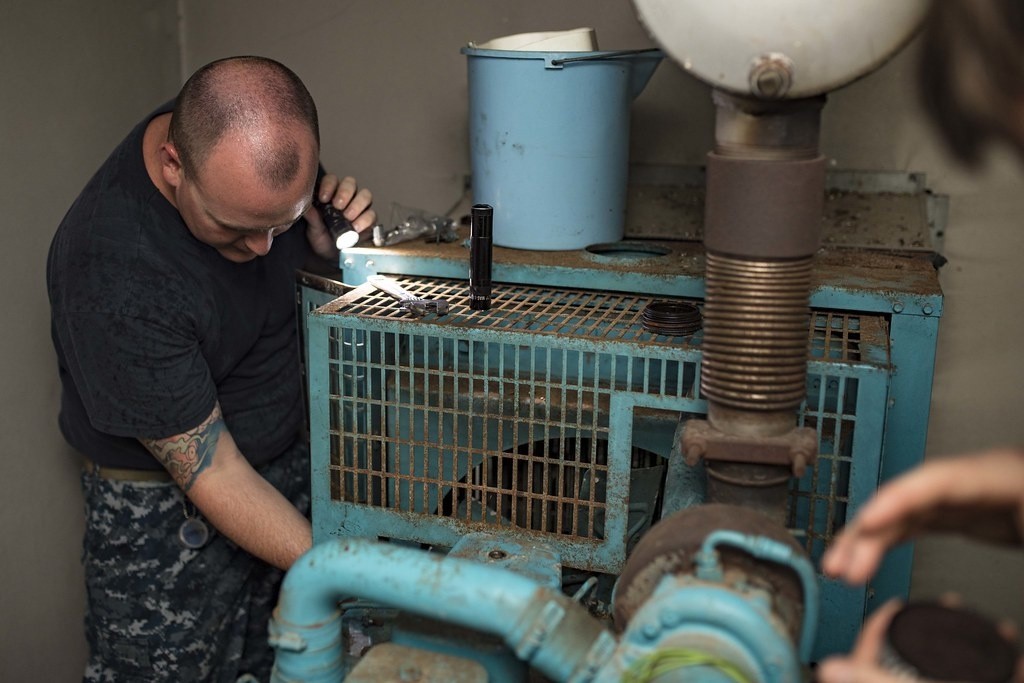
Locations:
[814,453,1024,683]
[46,55,377,683]
